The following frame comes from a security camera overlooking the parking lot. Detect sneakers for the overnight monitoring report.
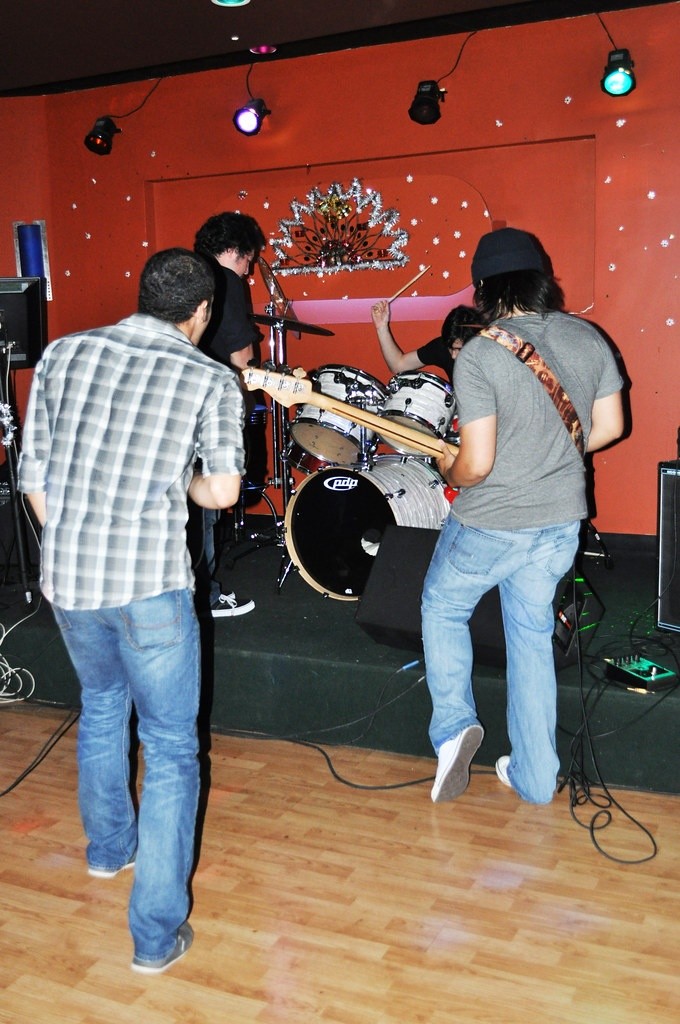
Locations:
[197,592,255,618]
[131,921,194,973]
[87,849,136,878]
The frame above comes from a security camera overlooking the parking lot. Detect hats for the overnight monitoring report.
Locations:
[470,229,553,285]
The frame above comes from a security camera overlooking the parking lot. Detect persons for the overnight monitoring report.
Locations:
[192,212,268,617]
[419,227,624,805]
[371,299,488,387]
[16,248,246,974]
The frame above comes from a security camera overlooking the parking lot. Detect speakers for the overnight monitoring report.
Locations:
[654,460,680,633]
[353,524,606,673]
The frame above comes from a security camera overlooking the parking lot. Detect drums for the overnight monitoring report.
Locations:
[380,369,459,457]
[282,453,453,604]
[287,363,391,464]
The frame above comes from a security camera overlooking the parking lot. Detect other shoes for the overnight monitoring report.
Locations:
[495,756,514,789]
[430,725,484,802]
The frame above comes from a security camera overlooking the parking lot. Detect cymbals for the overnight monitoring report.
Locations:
[257,256,301,340]
[251,312,335,337]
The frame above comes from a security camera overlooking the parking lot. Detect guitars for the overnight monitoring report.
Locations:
[241,366,462,463]
